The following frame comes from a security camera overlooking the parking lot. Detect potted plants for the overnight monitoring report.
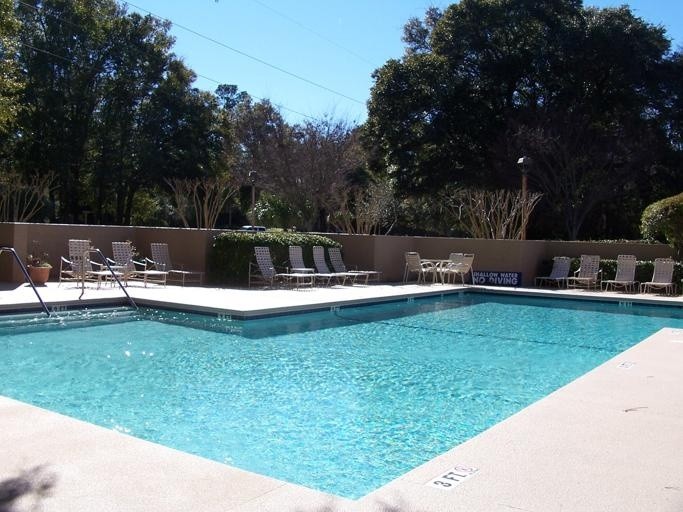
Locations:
[27,240,52,286]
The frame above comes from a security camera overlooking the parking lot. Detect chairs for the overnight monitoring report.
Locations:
[249,246,382,290]
[534,255,677,297]
[402,252,474,286]
[58,240,205,289]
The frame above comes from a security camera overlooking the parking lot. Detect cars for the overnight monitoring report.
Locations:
[237,225,266,232]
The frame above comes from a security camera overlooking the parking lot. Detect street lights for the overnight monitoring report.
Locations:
[248,170,257,230]
[517,155,532,240]
